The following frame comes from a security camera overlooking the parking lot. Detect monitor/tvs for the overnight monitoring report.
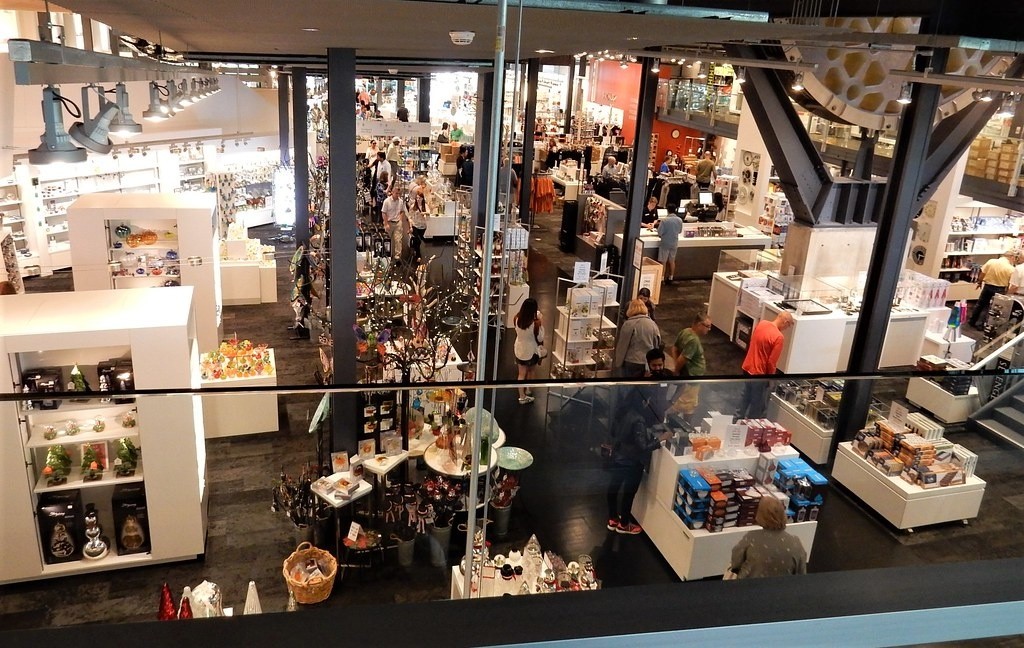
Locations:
[610,191,626,206]
[698,191,713,205]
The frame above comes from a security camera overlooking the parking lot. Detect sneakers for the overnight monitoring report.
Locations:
[616,523,642,534]
[607,516,621,531]
[519,390,535,404]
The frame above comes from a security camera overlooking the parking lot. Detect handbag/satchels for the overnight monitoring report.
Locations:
[600,443,613,472]
[437,134,450,143]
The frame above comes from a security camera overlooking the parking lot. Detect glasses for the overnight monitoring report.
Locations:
[700,322,711,330]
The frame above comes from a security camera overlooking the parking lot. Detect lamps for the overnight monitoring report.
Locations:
[576,50,804,91]
[28,78,221,164]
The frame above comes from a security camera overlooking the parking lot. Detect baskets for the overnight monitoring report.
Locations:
[283,542,337,604]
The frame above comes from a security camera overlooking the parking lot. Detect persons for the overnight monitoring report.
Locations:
[660,156,672,177]
[355,87,410,123]
[357,136,431,267]
[518,108,546,141]
[606,387,673,534]
[968,251,1024,332]
[435,122,463,167]
[731,496,807,579]
[601,156,616,177]
[552,90,561,109]
[307,99,328,131]
[641,197,683,286]
[454,144,518,215]
[513,298,545,404]
[684,192,725,222]
[695,151,717,189]
[733,310,792,425]
[616,287,711,429]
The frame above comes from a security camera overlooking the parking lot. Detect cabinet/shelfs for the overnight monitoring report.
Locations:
[0,286,209,585]
[543,267,626,435]
[715,175,794,249]
[397,136,530,331]
[66,193,224,368]
[938,194,1024,301]
[0,132,317,282]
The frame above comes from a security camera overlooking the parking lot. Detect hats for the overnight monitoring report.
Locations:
[392,137,402,141]
